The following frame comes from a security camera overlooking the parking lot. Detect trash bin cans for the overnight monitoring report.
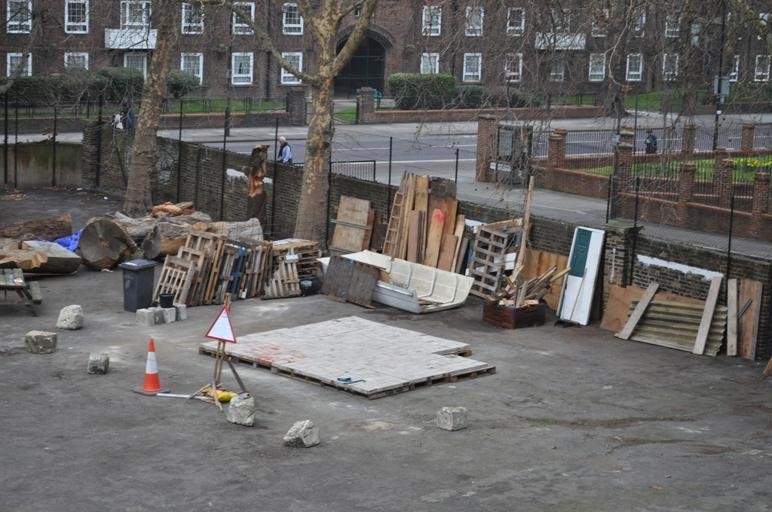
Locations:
[118,258,158,313]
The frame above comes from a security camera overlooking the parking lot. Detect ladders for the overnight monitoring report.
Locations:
[382,191,405,257]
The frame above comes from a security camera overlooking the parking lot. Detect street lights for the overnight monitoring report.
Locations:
[737,33,762,83]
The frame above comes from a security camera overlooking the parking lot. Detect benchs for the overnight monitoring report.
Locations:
[28,280,43,305]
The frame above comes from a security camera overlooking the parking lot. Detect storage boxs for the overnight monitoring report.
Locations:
[481,301,547,330]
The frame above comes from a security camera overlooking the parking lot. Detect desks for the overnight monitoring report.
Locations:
[0,269,38,317]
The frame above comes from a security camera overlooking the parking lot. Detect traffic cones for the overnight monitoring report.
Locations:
[133,337,170,397]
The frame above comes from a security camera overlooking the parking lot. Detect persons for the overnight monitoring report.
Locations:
[278,135,292,164]
[643,130,658,154]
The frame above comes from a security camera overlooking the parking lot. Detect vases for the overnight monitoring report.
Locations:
[159,294,175,308]
